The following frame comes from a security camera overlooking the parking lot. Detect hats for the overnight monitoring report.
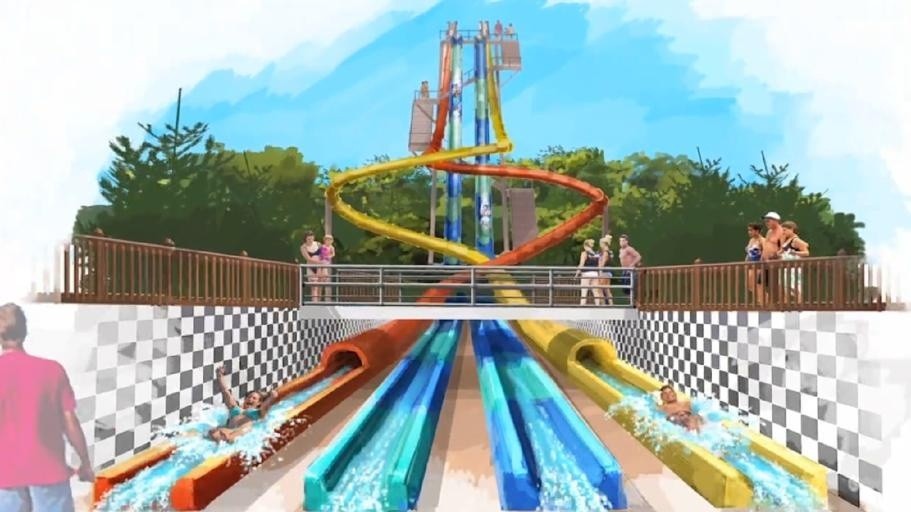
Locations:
[762,211,781,221]
